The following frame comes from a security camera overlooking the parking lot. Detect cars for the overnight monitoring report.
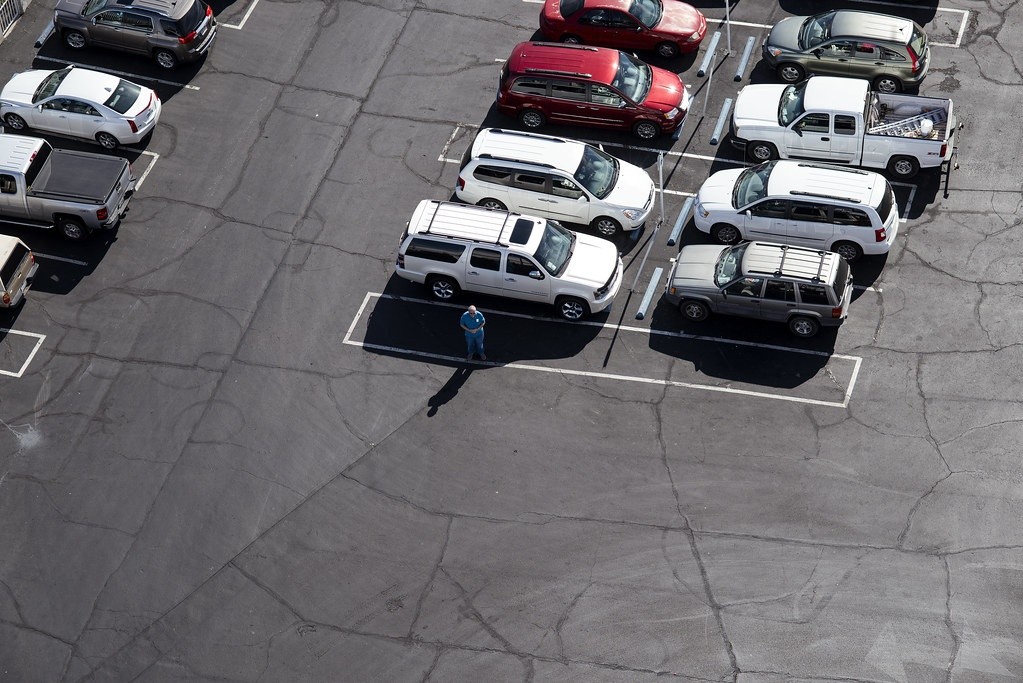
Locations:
[0,65,162,148]
[539,0,708,59]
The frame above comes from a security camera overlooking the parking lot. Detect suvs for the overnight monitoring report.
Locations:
[396,199,624,320]
[0,234,40,309]
[665,241,854,336]
[779,0,940,28]
[496,41,690,142]
[53,0,218,70]
[762,9,932,93]
[693,158,899,261]
[456,127,657,238]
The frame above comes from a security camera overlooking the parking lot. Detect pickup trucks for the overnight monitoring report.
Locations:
[729,76,962,189]
[0,133,136,244]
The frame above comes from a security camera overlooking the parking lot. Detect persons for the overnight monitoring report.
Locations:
[460,305,487,360]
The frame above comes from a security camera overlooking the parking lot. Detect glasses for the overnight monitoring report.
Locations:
[470,313,475,314]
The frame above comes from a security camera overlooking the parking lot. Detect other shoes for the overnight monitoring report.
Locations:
[468,354,472,360]
[480,354,486,360]
